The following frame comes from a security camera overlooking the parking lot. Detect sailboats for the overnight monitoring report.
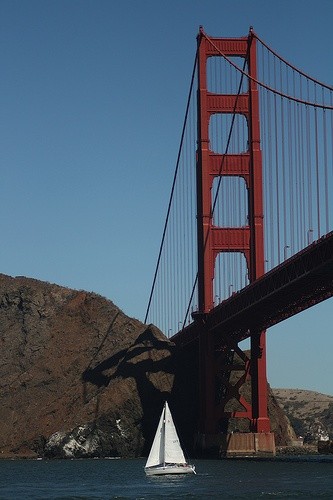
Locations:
[143,401,197,475]
